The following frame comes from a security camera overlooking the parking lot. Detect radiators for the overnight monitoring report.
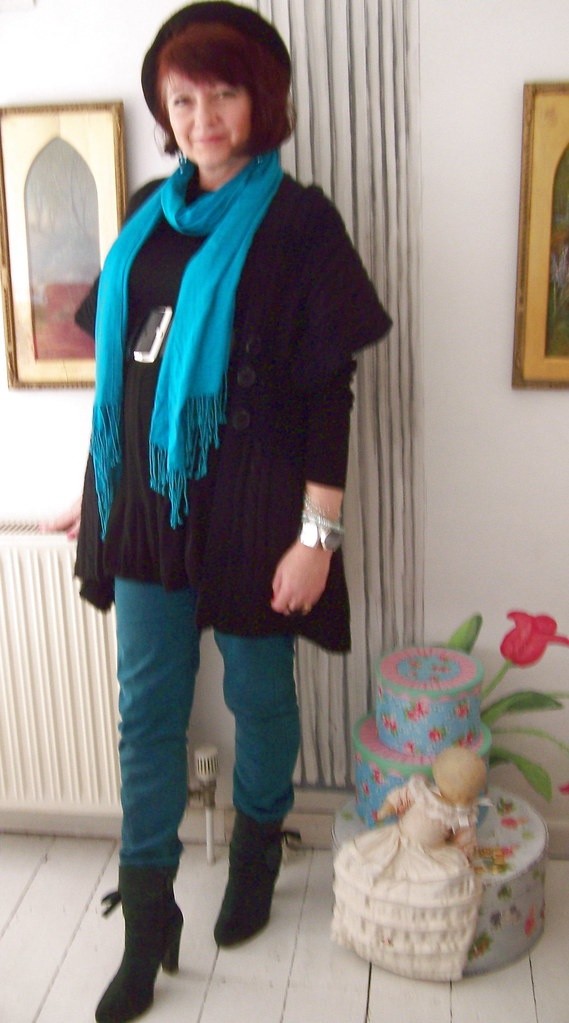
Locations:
[0,521,123,814]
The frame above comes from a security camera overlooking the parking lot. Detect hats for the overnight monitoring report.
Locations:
[141,0,293,127]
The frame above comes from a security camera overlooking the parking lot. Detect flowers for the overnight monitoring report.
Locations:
[451,611,569,806]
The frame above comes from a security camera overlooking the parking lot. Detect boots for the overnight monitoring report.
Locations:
[213,809,302,945]
[95,865,183,1023]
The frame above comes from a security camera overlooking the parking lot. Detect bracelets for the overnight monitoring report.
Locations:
[300,488,345,552]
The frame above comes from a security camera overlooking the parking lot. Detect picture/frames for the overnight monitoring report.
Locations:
[511,83,568,392]
[0,100,127,389]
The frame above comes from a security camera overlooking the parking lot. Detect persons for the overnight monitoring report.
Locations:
[39,0,394,1023]
[331,746,487,982]
